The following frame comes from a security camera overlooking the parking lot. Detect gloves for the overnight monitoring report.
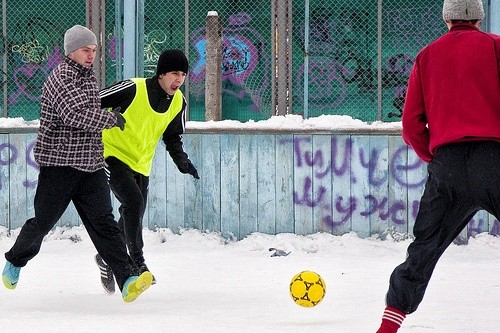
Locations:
[111,107,126,130]
[176,161,200,180]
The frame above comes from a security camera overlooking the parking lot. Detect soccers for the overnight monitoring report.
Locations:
[289,270,327,308]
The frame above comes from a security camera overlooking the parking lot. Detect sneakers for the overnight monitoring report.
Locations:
[94,252,115,294]
[138,262,157,285]
[121,270,153,303]
[2,260,21,290]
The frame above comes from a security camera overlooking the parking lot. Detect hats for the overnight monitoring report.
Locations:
[157,49,188,76]
[442,0,484,20]
[64,25,98,54]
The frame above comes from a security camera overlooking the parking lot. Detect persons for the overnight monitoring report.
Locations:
[2,24,153,304]
[375,0,500,333]
[94,48,201,294]
